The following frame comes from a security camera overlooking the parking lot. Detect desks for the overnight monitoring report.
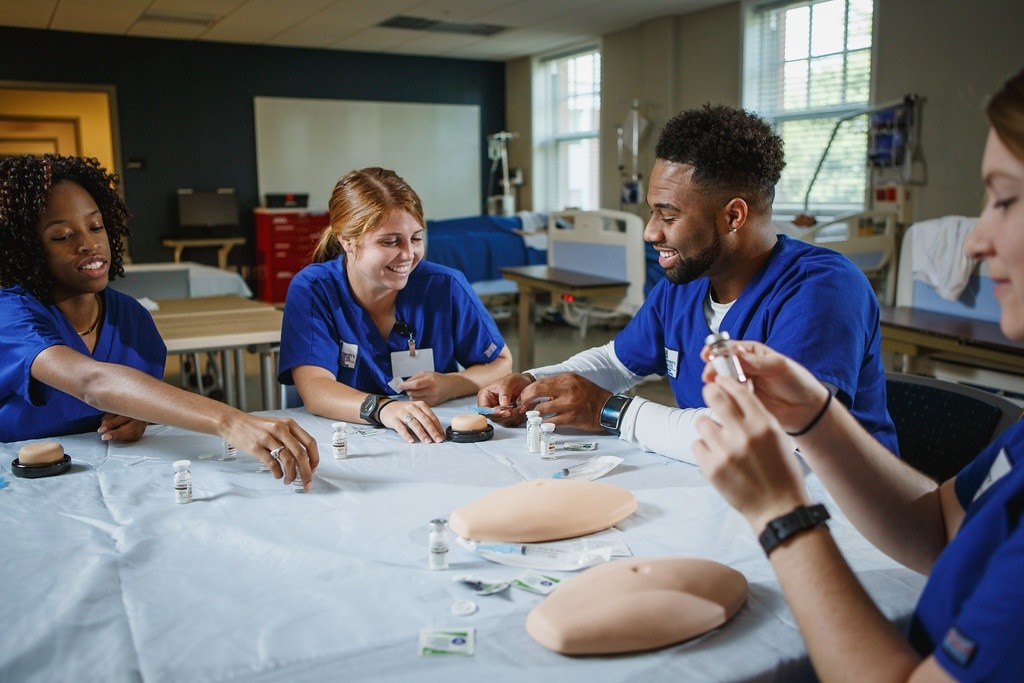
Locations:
[500,266,631,368]
[878,307,1024,390]
[161,239,245,271]
[109,264,284,412]
[0,396,926,683]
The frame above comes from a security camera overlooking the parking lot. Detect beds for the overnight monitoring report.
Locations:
[420,207,1024,409]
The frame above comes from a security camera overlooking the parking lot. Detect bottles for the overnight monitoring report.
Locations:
[428,519,450,570]
[221,439,237,461]
[173,459,193,504]
[525,410,556,459]
[704,331,747,384]
[330,421,348,460]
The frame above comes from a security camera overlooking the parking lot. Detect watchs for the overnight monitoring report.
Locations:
[360,394,391,429]
[598,393,634,437]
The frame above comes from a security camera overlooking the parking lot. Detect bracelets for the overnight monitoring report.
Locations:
[785,387,833,436]
[758,502,831,559]
[378,399,399,424]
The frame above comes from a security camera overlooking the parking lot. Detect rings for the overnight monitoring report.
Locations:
[403,414,413,425]
[271,446,285,459]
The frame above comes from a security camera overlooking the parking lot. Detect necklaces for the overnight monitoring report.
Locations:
[77,294,102,336]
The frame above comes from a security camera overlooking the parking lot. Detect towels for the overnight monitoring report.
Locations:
[910,213,982,301]
[517,209,548,250]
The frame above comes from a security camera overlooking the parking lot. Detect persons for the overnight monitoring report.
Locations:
[277,166,513,444]
[0,151,320,493]
[477,101,901,461]
[692,67,1024,683]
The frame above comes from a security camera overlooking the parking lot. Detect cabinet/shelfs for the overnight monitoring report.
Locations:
[254,208,332,307]
